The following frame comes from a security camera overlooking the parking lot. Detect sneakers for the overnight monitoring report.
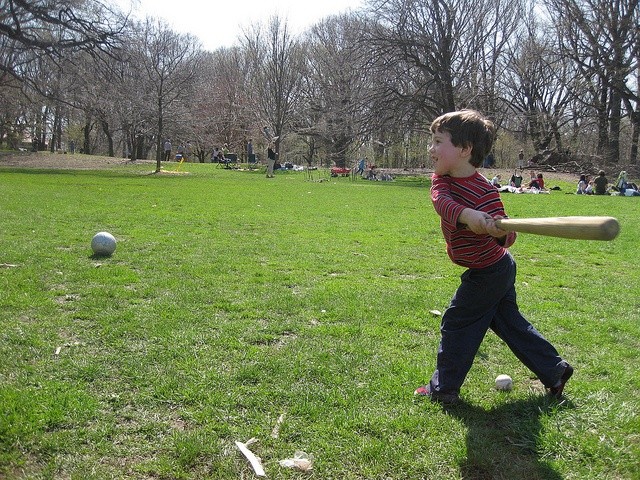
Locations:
[414,387,459,403]
[549,363,573,399]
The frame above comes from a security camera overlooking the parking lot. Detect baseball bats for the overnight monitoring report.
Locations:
[464,216,620,241]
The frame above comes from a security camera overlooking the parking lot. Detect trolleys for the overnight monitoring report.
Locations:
[331,160,353,178]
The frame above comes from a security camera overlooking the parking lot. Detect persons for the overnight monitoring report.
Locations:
[577,170,640,196]
[247,139,252,157]
[217,151,232,163]
[492,175,502,188]
[176,143,185,153]
[529,170,536,180]
[164,139,171,161]
[518,150,524,170]
[510,171,523,187]
[412,108,573,401]
[186,141,192,163]
[266,141,278,178]
[528,173,544,189]
[367,166,394,182]
[355,156,366,175]
[69,140,75,154]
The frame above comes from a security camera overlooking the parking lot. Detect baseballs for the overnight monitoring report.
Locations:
[495,374,513,390]
[91,231,116,255]
